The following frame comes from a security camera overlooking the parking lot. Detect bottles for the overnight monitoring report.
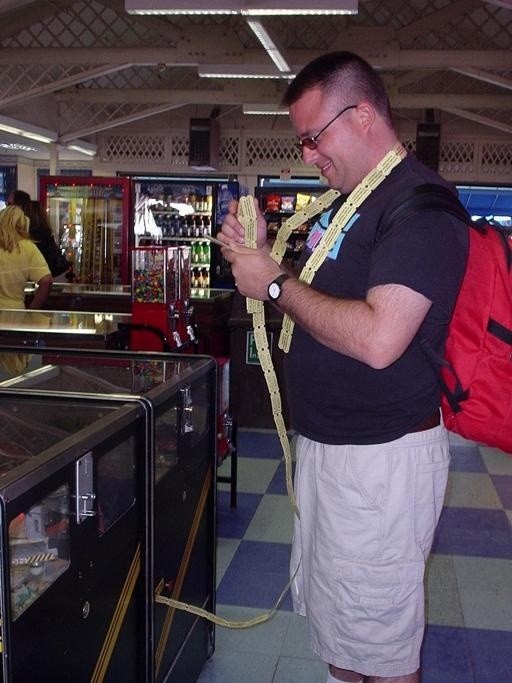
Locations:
[155,188,214,288]
[216,183,235,224]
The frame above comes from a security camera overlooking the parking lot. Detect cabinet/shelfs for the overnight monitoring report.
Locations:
[255,186,329,264]
[0,345,219,683]
[129,175,240,289]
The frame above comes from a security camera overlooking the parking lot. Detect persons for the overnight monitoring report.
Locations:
[0,204,54,309]
[5,190,30,205]
[1,310,50,376]
[216,50,470,682]
[22,199,73,283]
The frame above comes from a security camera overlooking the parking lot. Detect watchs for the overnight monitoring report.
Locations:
[268,274,291,307]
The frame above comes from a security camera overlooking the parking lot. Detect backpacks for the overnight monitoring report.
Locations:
[371,187,512,454]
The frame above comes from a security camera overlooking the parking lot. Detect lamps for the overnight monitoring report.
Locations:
[0,113,57,144]
[124,0,359,115]
[67,139,98,157]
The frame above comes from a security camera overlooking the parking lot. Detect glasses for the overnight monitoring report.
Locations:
[293,104,357,153]
